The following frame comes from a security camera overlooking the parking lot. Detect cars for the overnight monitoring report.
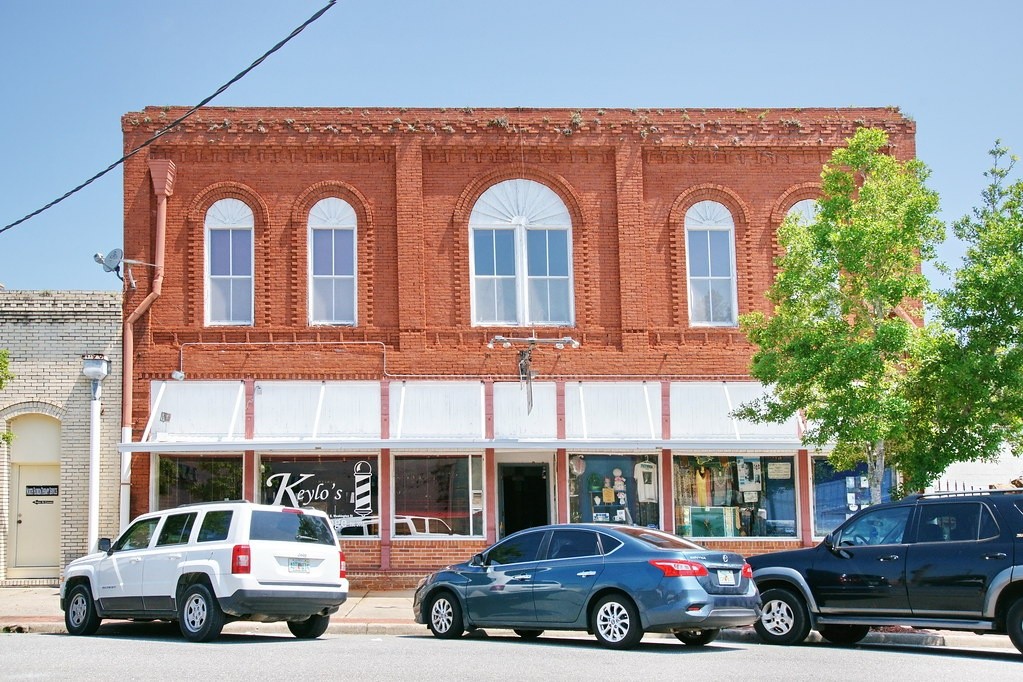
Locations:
[413,522,762,650]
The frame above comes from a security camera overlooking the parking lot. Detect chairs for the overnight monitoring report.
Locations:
[919,524,944,542]
[979,517,995,541]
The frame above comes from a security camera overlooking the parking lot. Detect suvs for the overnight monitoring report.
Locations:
[61,500,350,642]
[743,486,1023,655]
[335,514,459,537]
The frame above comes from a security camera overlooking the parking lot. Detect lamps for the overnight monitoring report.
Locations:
[486,334,580,350]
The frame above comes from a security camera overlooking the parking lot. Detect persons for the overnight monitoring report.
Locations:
[755,474,760,483]
[643,472,650,484]
[741,461,754,484]
